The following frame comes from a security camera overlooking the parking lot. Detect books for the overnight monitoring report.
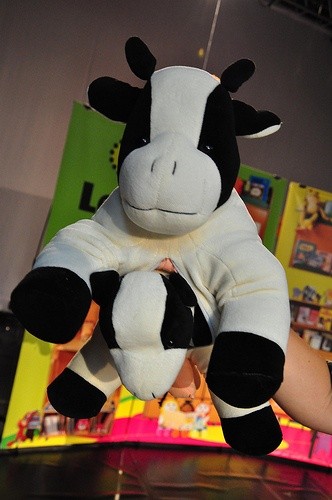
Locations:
[285,239,332,355]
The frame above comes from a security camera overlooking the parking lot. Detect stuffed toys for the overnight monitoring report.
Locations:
[9,35,291,458]
[293,187,332,231]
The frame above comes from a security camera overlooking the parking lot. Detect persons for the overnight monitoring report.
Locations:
[155,257,332,436]
